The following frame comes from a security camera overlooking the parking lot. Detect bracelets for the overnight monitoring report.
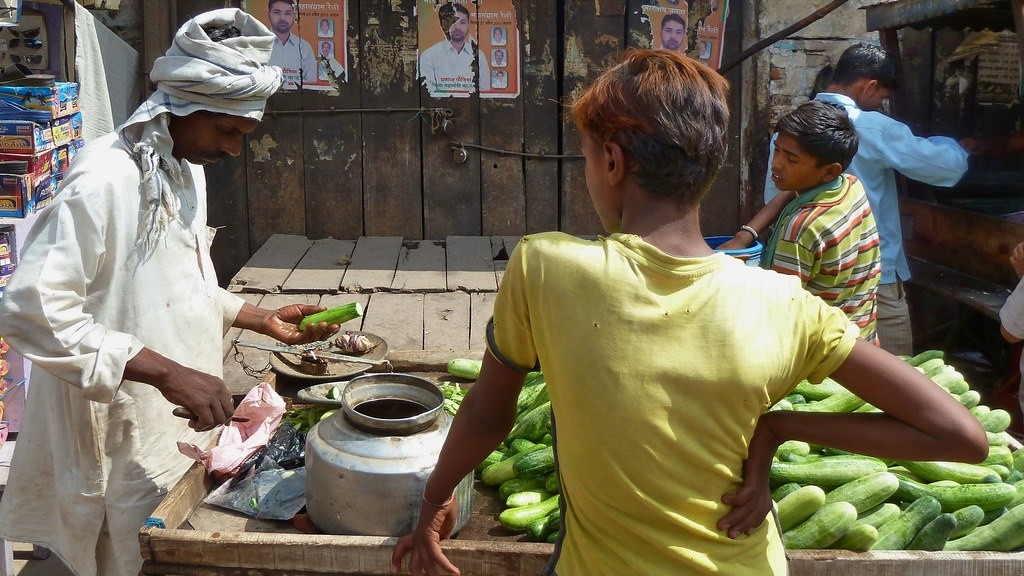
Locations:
[422,487,456,507]
[740,224,758,240]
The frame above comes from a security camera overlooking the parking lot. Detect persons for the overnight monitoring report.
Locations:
[660,13,686,51]
[419,3,490,91]
[319,20,333,36]
[319,41,334,57]
[959,130,1024,159]
[760,41,970,359]
[0,7,342,575]
[699,41,707,57]
[491,49,507,66]
[393,46,989,576]
[490,28,507,45]
[715,97,881,349]
[492,70,507,88]
[266,0,316,84]
[997,239,1024,414]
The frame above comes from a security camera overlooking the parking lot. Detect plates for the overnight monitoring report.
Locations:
[296,381,348,405]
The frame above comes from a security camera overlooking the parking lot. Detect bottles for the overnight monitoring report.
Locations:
[305,373,471,536]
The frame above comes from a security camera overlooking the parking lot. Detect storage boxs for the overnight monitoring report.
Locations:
[50,115,74,148]
[54,172,68,185]
[0,85,73,124]
[69,111,84,141]
[0,233,11,267]
[137,349,1024,576]
[0,264,17,301]
[32,176,60,214]
[0,173,33,220]
[0,119,55,155]
[0,150,52,181]
[55,81,81,114]
[50,145,69,176]
[946,33,1023,107]
[67,137,85,167]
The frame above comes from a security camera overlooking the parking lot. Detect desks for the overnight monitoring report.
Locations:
[219,291,498,406]
[905,261,1013,375]
[225,230,605,292]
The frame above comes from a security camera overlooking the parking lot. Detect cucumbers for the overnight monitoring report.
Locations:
[300,302,363,332]
[443,358,561,542]
[767,350,1024,550]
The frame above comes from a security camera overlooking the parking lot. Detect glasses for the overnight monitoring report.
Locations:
[6,27,40,38]
[9,39,43,49]
[9,55,43,65]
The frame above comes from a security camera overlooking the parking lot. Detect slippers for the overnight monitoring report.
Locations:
[0,63,56,88]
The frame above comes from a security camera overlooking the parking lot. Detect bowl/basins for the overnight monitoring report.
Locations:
[703,236,762,267]
